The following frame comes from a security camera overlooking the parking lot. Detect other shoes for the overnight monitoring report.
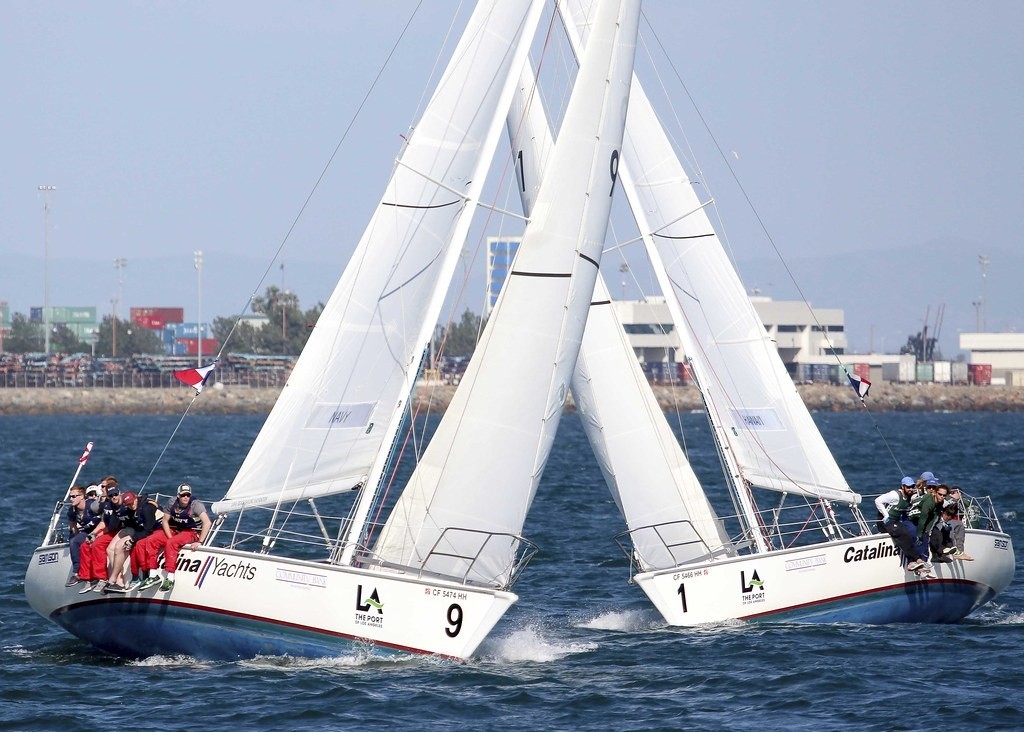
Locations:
[107,584,125,592]
[69,575,83,586]
[99,582,115,595]
[907,546,975,580]
[125,578,141,592]
[159,578,175,592]
[93,580,109,593]
[139,575,162,590]
[79,580,98,594]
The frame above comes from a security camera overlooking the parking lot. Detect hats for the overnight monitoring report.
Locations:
[901,476,916,486]
[98,484,103,495]
[176,484,192,498]
[86,486,99,496]
[921,472,939,481]
[107,486,119,496]
[926,480,938,486]
[121,492,136,506]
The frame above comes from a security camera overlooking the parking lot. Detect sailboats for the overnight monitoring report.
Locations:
[25,1,652,663]
[502,1,1018,633]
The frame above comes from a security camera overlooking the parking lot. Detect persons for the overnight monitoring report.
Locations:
[437,351,470,386]
[101,492,137,594]
[65,476,119,594]
[0,347,287,389]
[137,482,211,593]
[122,492,165,593]
[874,471,974,579]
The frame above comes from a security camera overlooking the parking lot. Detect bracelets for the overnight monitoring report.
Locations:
[198,541,203,545]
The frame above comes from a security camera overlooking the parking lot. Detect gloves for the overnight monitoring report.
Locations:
[122,539,135,552]
[86,533,97,545]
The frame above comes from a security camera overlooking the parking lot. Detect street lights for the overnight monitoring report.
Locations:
[112,255,128,321]
[278,261,287,355]
[978,254,990,333]
[970,300,982,334]
[36,184,58,353]
[193,249,205,368]
[109,299,122,357]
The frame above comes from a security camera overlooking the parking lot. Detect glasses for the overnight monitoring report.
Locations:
[109,493,120,498]
[927,485,937,490]
[178,494,191,498]
[69,494,83,498]
[87,492,97,497]
[904,485,915,489]
[101,485,107,488]
[937,492,947,497]
